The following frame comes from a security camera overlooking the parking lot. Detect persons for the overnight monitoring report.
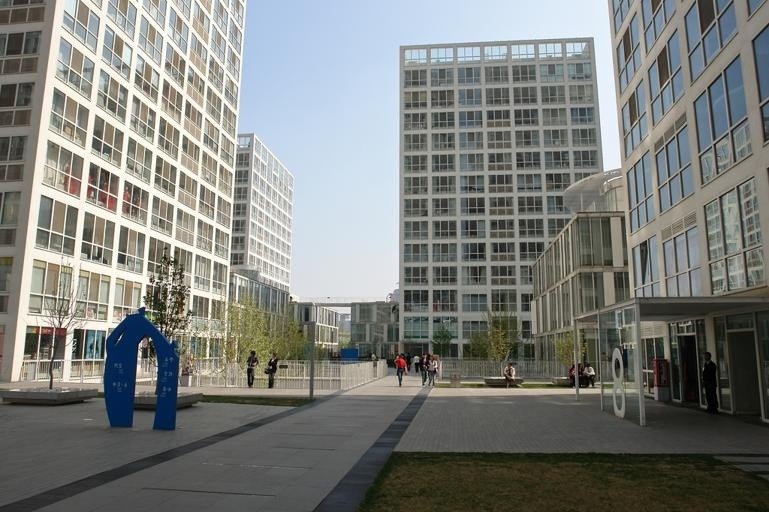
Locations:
[370,350,377,361]
[244,349,259,386]
[701,350,719,414]
[578,363,584,388]
[583,363,595,388]
[570,364,575,388]
[267,351,278,388]
[503,362,516,390]
[394,350,438,387]
